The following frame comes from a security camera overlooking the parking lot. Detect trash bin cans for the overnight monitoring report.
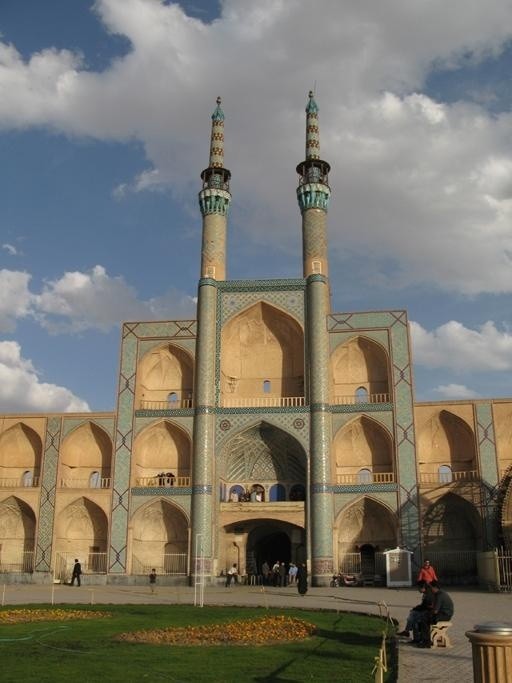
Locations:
[465,621,512,683]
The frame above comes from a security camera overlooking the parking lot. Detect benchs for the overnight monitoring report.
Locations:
[430,621,454,648]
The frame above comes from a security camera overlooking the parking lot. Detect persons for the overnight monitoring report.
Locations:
[155,472,175,485]
[230,489,261,502]
[69,559,82,587]
[396,559,453,647]
[225,564,239,587]
[261,561,305,587]
[149,568,157,592]
